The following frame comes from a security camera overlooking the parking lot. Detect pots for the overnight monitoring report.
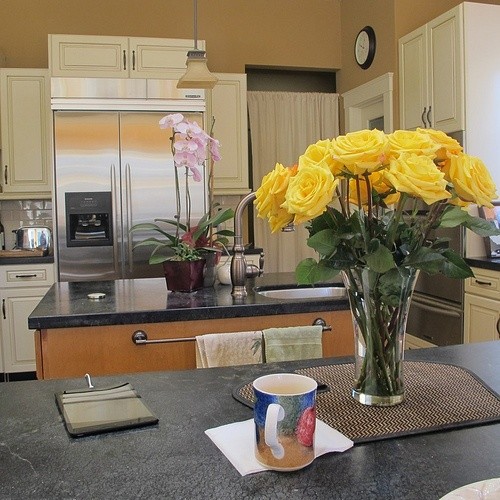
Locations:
[13,225,53,257]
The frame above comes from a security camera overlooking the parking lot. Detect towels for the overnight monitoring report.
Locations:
[262,325,322,363]
[195,331,262,369]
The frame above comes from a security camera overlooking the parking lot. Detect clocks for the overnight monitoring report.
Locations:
[355,25,376,70]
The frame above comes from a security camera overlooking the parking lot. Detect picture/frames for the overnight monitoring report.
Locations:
[477,201,500,256]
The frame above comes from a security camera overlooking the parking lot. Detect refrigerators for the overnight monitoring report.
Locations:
[50,97,213,282]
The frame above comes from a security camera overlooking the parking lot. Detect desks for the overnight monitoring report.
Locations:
[0,343,500,500]
[28,272,353,381]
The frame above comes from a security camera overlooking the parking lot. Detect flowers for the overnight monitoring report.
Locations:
[129,113,235,267]
[253,128,498,396]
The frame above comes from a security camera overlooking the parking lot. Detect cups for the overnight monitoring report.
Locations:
[252,373,318,471]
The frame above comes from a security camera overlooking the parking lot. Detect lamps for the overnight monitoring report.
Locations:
[177,0,219,89]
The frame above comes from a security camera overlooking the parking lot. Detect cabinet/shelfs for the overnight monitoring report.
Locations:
[397,2,500,135]
[0,263,55,382]
[0,34,252,200]
[464,267,500,344]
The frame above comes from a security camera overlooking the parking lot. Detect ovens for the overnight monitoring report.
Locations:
[397,130,466,348]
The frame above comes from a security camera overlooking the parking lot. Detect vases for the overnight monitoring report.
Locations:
[340,265,421,406]
[198,252,217,288]
[163,259,206,292]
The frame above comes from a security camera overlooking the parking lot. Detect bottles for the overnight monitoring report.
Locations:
[0,222,5,250]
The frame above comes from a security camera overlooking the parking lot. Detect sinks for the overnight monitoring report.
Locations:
[257,287,346,299]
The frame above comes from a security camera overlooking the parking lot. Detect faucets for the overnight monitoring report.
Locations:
[230,191,295,296]
